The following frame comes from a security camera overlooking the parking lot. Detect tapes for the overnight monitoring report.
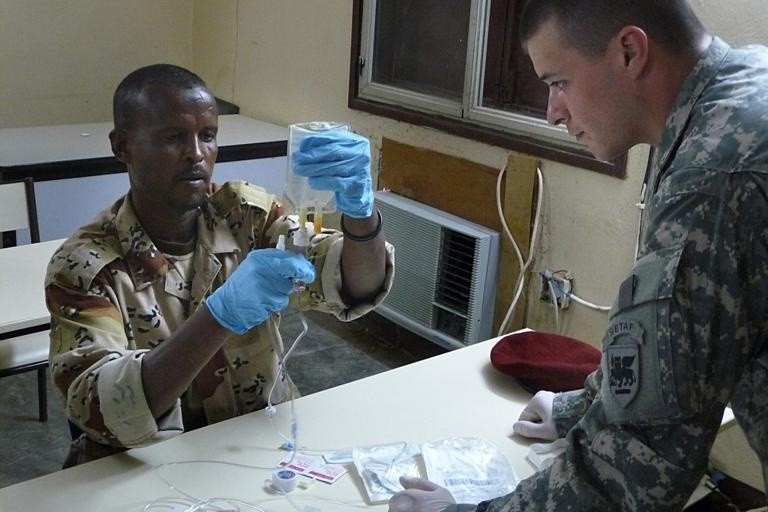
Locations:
[272,469,297,492]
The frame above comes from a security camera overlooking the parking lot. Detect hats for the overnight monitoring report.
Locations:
[490,332,602,394]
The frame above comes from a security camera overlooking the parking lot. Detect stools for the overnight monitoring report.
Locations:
[0,328,53,422]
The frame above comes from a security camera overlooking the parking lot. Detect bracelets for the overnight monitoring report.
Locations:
[341,209,382,243]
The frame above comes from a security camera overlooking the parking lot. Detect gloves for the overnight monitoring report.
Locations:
[513,390,558,441]
[388,475,454,512]
[206,248,315,334]
[291,131,375,218]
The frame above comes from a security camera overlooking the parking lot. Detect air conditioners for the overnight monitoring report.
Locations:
[363,188,499,351]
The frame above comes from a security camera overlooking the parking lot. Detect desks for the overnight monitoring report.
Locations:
[1,327,713,512]
[0,238,76,341]
[0,113,289,244]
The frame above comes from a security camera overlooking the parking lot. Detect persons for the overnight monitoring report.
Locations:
[388,0,768,511]
[44,63,396,470]
